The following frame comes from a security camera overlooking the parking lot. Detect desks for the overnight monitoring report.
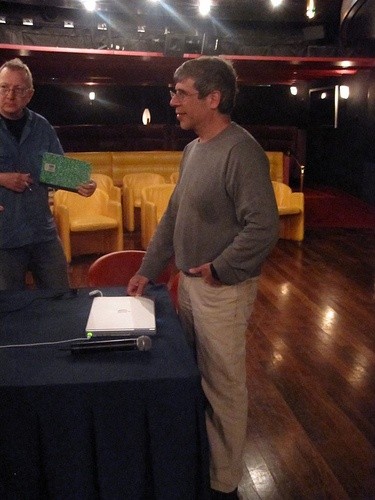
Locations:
[0,285,202,499]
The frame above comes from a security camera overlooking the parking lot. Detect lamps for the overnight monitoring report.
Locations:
[142,108,152,131]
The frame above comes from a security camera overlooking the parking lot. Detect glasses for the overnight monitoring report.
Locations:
[0,86,29,93]
[170,90,210,97]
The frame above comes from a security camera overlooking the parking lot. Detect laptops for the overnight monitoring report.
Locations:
[84,296,157,336]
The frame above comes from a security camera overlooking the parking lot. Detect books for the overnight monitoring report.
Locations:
[40,151,91,191]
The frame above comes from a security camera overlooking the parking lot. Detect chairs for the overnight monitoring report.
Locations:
[141,184,177,250]
[123,172,167,232]
[53,173,124,264]
[88,250,175,292]
[268,181,304,241]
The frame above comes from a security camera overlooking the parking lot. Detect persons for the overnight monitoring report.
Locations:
[127,56,282,500]
[0,58,98,290]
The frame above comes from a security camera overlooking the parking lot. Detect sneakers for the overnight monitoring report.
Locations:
[210,487,240,500]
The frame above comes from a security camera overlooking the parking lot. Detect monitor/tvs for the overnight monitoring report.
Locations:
[308,84,339,130]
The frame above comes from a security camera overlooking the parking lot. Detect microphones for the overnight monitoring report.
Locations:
[64,335,152,355]
